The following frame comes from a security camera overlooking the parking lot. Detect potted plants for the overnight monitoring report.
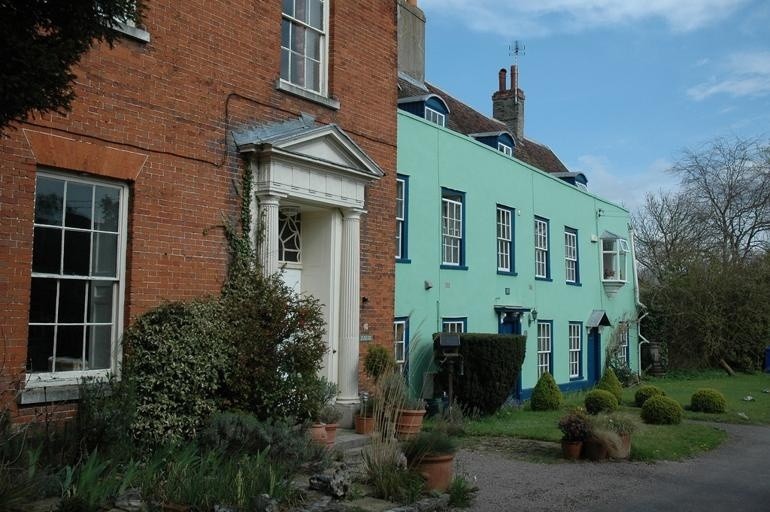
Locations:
[312,376,341,444]
[392,399,427,441]
[606,418,635,457]
[402,431,458,492]
[321,406,344,445]
[559,415,588,459]
[354,397,378,434]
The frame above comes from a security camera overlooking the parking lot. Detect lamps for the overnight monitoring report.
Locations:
[528,308,537,327]
